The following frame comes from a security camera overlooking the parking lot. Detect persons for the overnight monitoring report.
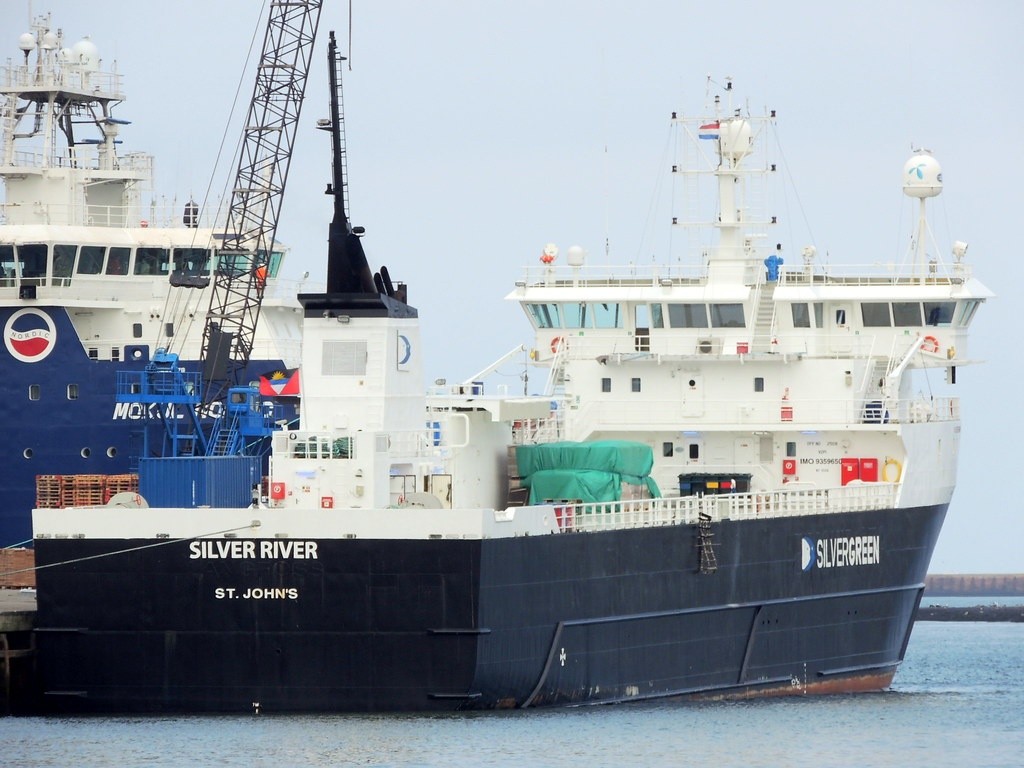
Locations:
[257,264,266,298]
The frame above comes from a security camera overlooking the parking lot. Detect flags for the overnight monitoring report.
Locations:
[260,368,299,395]
[699,124,718,139]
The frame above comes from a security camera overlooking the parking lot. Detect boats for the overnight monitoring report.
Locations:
[0,0,996,714]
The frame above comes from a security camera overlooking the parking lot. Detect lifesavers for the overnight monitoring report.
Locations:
[550,335,567,355]
[921,334,940,355]
[882,459,902,483]
